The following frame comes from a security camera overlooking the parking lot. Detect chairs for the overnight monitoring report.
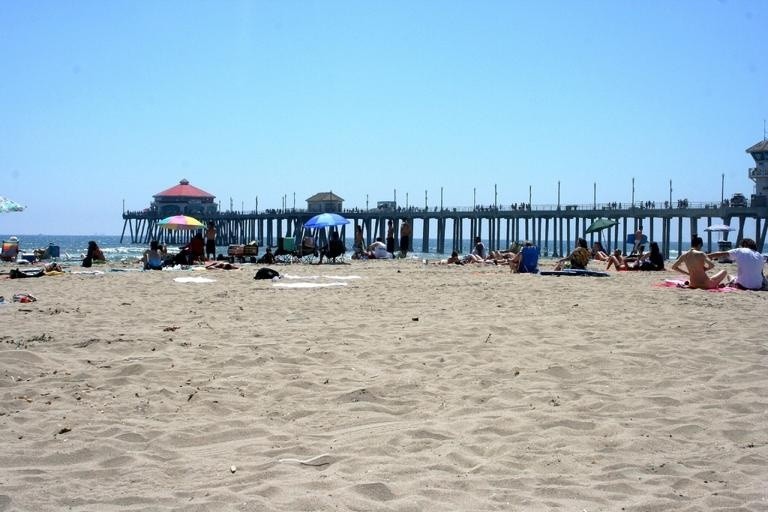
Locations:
[510,246,538,273]
[38,246,59,262]
[0,240,19,262]
[272,238,346,265]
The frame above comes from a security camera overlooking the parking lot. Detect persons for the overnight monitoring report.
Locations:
[366,237,386,259]
[142,221,216,272]
[385,220,396,259]
[318,231,346,264]
[398,217,411,257]
[352,224,365,258]
[86,240,107,262]
[261,247,274,264]
[669,233,727,289]
[429,226,667,273]
[32,242,54,255]
[45,262,62,272]
[707,238,768,291]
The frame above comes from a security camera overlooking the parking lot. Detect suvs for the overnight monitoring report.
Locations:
[729,192,749,207]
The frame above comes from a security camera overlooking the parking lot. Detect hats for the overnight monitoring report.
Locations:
[7,237,19,242]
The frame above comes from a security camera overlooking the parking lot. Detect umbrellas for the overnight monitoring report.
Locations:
[703,224,735,240]
[0,196,26,215]
[583,219,620,252]
[155,214,208,245]
[301,212,349,248]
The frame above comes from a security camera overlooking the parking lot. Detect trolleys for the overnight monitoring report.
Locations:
[226,242,261,264]
